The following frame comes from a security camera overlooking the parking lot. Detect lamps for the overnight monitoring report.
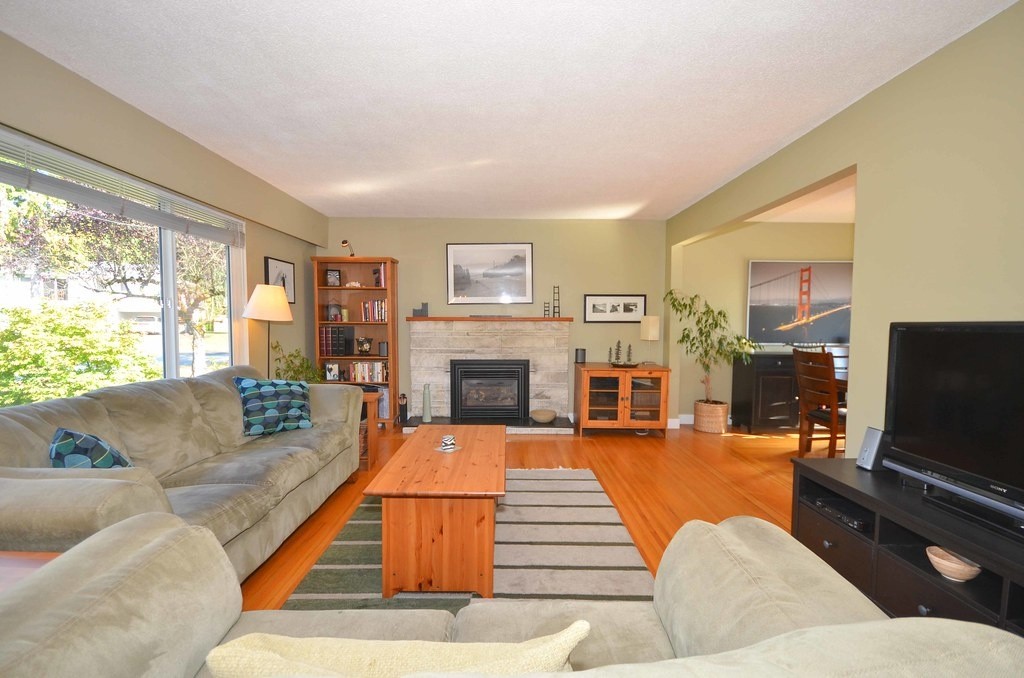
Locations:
[242,283,294,379]
[341,240,355,257]
[639,315,660,365]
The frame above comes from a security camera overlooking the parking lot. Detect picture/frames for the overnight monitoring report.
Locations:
[446,242,534,305]
[324,268,341,287]
[745,259,852,347]
[583,293,647,323]
[264,256,296,305]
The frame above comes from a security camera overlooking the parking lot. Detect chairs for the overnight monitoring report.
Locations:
[793,348,846,458]
[822,345,849,381]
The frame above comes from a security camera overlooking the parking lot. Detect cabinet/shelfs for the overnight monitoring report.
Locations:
[573,362,672,438]
[309,255,399,437]
[730,351,801,434]
[789,457,1024,637]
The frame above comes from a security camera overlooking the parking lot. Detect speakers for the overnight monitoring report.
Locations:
[856,428,889,471]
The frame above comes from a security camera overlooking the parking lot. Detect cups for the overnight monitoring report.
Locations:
[576,349,586,362]
[355,338,373,355]
[332,314,341,321]
[341,308,349,321]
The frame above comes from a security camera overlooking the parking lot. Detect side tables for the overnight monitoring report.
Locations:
[0,550,61,590]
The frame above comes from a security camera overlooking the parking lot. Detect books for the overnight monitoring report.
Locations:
[320,263,387,356]
[324,360,389,382]
[378,387,390,418]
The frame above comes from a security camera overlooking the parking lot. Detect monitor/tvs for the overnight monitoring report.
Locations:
[882,321,1024,539]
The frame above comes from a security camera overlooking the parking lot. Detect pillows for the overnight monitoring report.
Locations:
[204,618,591,678]
[231,376,314,438]
[48,426,134,468]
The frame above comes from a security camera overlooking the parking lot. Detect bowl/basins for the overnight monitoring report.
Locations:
[926,546,982,583]
[530,409,557,423]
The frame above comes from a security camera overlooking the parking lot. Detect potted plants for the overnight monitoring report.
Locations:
[661,287,765,433]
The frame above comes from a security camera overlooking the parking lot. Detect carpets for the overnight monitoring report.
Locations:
[280,465,655,616]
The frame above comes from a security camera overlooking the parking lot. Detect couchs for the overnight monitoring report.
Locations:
[0,363,365,586]
[1,510,1024,678]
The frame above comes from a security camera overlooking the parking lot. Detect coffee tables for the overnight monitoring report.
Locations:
[363,423,507,598]
